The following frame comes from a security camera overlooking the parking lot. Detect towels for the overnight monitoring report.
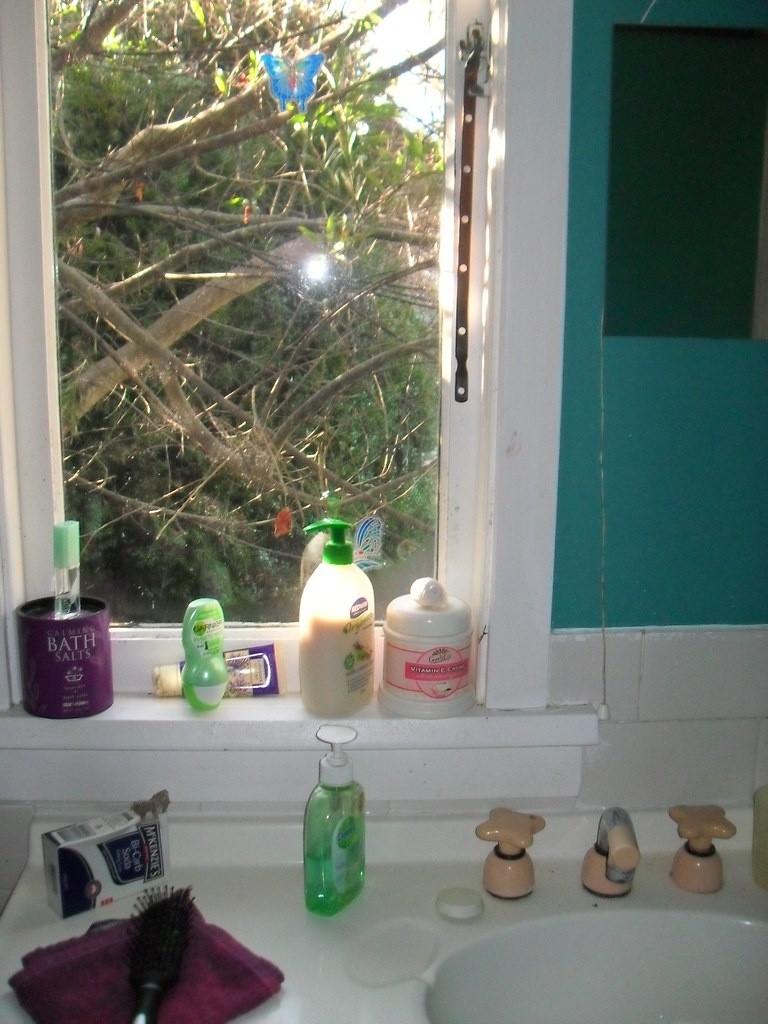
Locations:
[5,895,286,1023]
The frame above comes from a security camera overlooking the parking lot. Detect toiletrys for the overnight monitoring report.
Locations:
[300,725,371,916]
[152,642,294,702]
[180,595,233,712]
[297,518,377,723]
[298,493,358,598]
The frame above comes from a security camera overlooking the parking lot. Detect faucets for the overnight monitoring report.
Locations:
[576,802,646,906]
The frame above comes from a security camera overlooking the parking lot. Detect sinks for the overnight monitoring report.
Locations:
[414,904,768,1023]
